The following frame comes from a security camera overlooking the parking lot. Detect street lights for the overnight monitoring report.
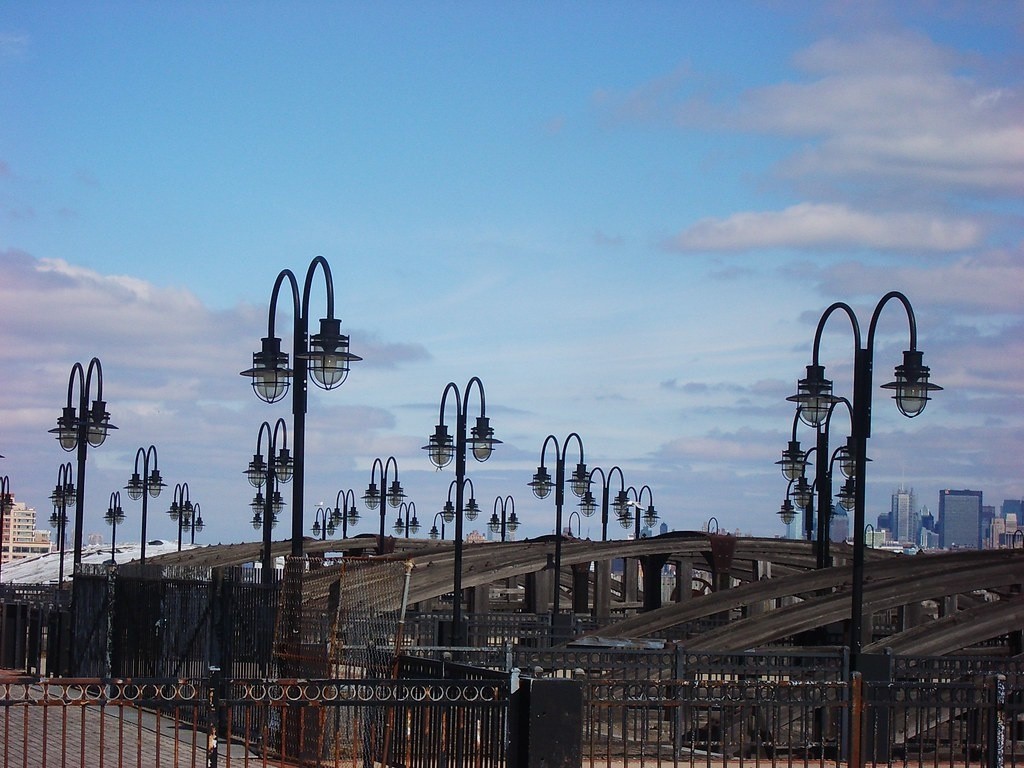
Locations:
[420,376,520,678]
[772,289,946,768]
[166,481,206,553]
[47,357,127,685]
[122,445,169,569]
[528,432,660,677]
[239,253,420,758]
